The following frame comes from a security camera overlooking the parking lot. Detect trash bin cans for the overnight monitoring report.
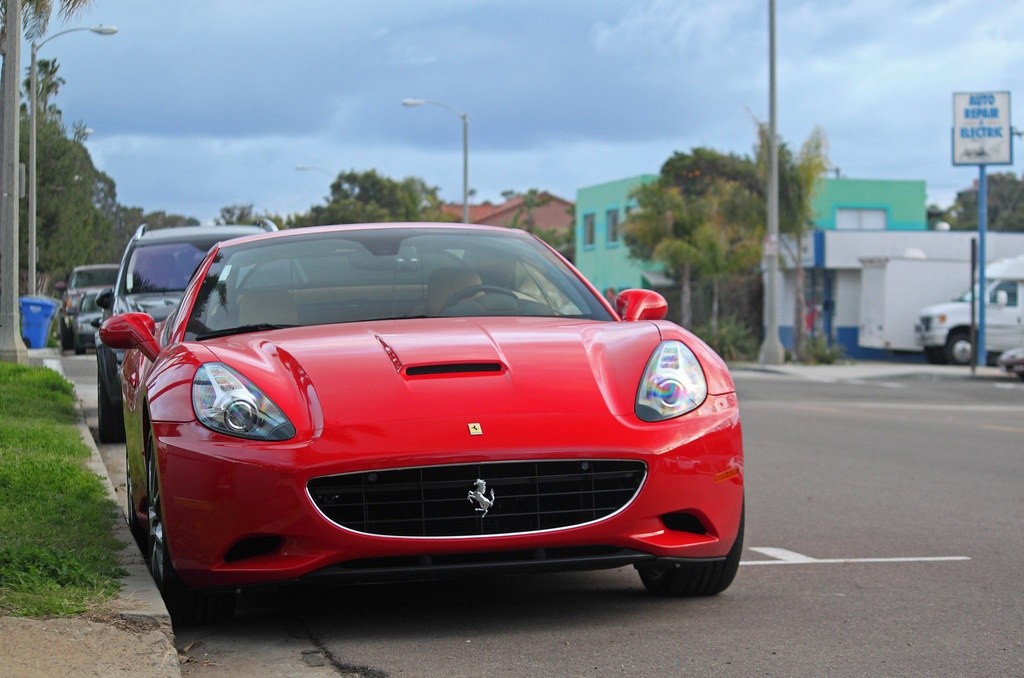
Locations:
[19,295,61,349]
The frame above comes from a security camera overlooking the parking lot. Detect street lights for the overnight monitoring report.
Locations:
[401,98,469,223]
[297,165,337,179]
[29,25,118,297]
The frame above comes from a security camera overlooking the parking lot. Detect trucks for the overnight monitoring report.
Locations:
[857,255,990,352]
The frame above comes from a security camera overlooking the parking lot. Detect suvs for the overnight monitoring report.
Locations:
[95,218,279,444]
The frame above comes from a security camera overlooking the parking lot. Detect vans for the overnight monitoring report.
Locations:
[913,256,1023,366]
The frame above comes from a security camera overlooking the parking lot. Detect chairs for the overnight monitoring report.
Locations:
[430,266,484,314]
[239,286,299,327]
[148,254,184,290]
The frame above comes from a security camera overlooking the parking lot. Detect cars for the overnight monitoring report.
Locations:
[54,264,120,350]
[997,347,1024,380]
[99,221,745,627]
[65,291,103,355]
